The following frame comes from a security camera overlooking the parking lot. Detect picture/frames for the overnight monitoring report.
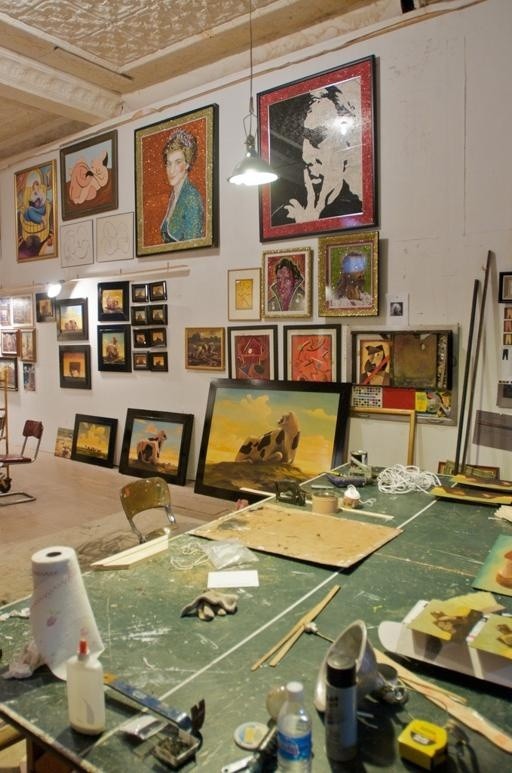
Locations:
[497,270,512,303]
[254,53,379,243]
[60,128,119,221]
[183,324,459,426]
[317,230,379,318]
[13,158,59,263]
[262,245,313,318]
[70,379,353,503]
[131,102,220,260]
[227,267,262,321]
[1,277,170,389]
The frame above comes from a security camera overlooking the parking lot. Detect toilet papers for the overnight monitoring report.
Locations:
[29,546,105,680]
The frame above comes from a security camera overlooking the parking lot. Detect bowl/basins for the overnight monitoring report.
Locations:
[311,618,378,713]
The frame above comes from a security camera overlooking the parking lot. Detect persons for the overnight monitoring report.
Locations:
[270,85,363,228]
[157,133,205,245]
[267,258,305,313]
[328,252,372,310]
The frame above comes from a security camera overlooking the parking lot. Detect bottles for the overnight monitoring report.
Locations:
[324,653,358,763]
[277,681,313,772]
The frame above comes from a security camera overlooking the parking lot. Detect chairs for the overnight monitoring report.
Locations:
[0,420,44,507]
[118,475,185,542]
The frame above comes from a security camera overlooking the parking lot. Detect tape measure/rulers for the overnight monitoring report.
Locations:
[397,719,448,771]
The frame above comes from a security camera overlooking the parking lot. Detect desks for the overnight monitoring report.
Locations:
[2,456,509,773]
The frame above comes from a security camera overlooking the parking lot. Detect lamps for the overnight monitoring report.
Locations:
[225,0,279,184]
